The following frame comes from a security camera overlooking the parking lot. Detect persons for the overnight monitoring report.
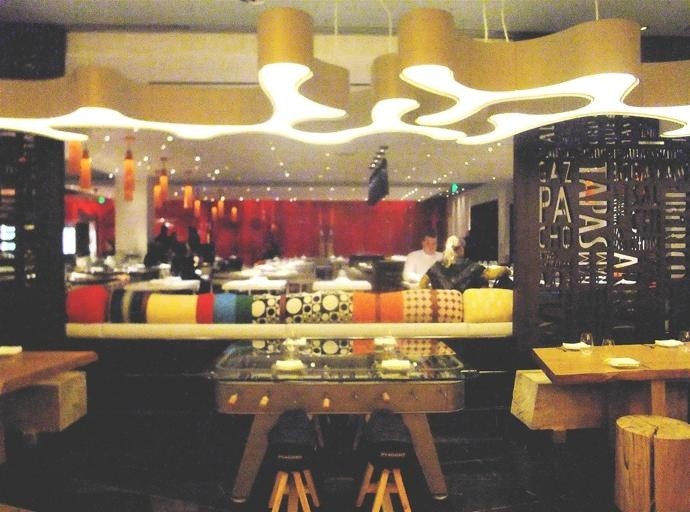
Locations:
[402,231,445,283]
[143,221,179,279]
[104,238,116,257]
[418,234,512,292]
[170,239,216,294]
[263,222,281,260]
[186,225,201,256]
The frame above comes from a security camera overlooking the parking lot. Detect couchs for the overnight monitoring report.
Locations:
[64,288,515,342]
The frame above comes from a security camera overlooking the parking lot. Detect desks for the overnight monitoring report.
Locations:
[0,342,102,466]
[208,343,466,511]
[64,247,515,290]
[536,339,690,436]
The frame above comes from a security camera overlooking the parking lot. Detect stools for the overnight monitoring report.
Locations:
[261,410,419,511]
[613,412,688,510]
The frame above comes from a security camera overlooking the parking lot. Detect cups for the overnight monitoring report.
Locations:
[579,332,594,356]
[598,338,616,360]
[681,330,690,352]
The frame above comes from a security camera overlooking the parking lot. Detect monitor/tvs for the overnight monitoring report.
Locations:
[62,226,78,256]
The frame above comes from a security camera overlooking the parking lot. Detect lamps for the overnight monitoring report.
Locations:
[0,0,686,225]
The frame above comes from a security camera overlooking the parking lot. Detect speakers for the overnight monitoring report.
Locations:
[0,22,66,81]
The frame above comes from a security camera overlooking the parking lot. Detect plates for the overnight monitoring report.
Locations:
[654,338,683,348]
[604,356,640,369]
[562,342,591,351]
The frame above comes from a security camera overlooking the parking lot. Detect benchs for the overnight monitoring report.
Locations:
[508,369,690,443]
